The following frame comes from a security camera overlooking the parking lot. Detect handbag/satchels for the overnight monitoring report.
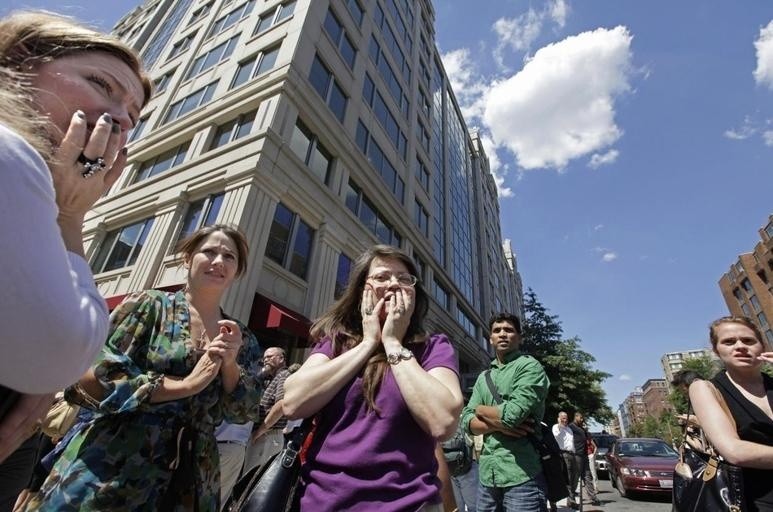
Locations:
[231,436,303,512]
[672,378,743,512]
[537,425,571,504]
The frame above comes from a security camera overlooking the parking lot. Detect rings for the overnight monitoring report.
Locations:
[78,153,106,179]
[399,305,405,313]
[366,309,371,315]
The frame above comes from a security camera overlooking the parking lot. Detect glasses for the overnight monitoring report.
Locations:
[362,272,420,289]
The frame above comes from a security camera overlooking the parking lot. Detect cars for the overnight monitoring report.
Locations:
[589,429,679,497]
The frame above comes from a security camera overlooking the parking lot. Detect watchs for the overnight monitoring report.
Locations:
[387,347,414,365]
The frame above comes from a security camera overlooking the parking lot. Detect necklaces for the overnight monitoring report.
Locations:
[199,323,208,348]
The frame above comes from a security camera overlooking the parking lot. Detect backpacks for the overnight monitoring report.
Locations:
[440,432,473,478]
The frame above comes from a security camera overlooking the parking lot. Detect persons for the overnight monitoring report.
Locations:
[457,312,550,510]
[281,244,461,512]
[670,370,708,454]
[538,411,600,509]
[212,348,301,511]
[0,8,152,396]
[436,397,484,510]
[42,225,264,511]
[689,316,773,512]
